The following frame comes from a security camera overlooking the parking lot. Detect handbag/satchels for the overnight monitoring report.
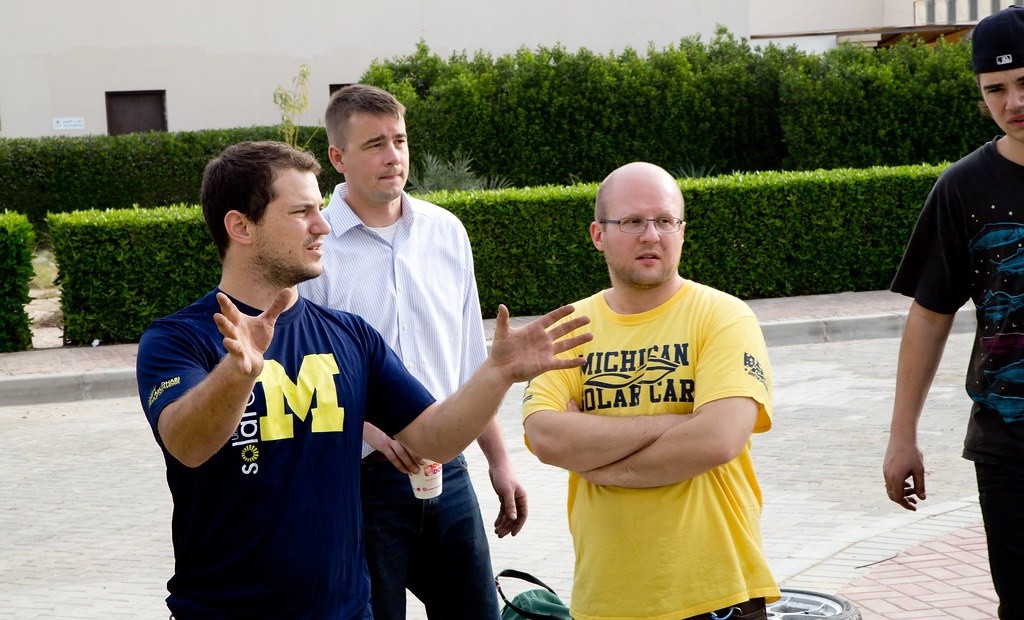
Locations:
[495,569,571,620]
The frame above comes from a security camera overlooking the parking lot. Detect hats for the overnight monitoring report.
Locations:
[971,5,1024,74]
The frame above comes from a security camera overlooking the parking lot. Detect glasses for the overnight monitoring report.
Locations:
[599,217,684,233]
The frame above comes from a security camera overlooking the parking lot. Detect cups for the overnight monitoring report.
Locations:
[409,452,443,499]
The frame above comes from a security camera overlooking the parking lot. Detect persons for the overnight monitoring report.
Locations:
[882,4,1024,620]
[296,84,529,620]
[522,162,781,620]
[137,141,593,620]
